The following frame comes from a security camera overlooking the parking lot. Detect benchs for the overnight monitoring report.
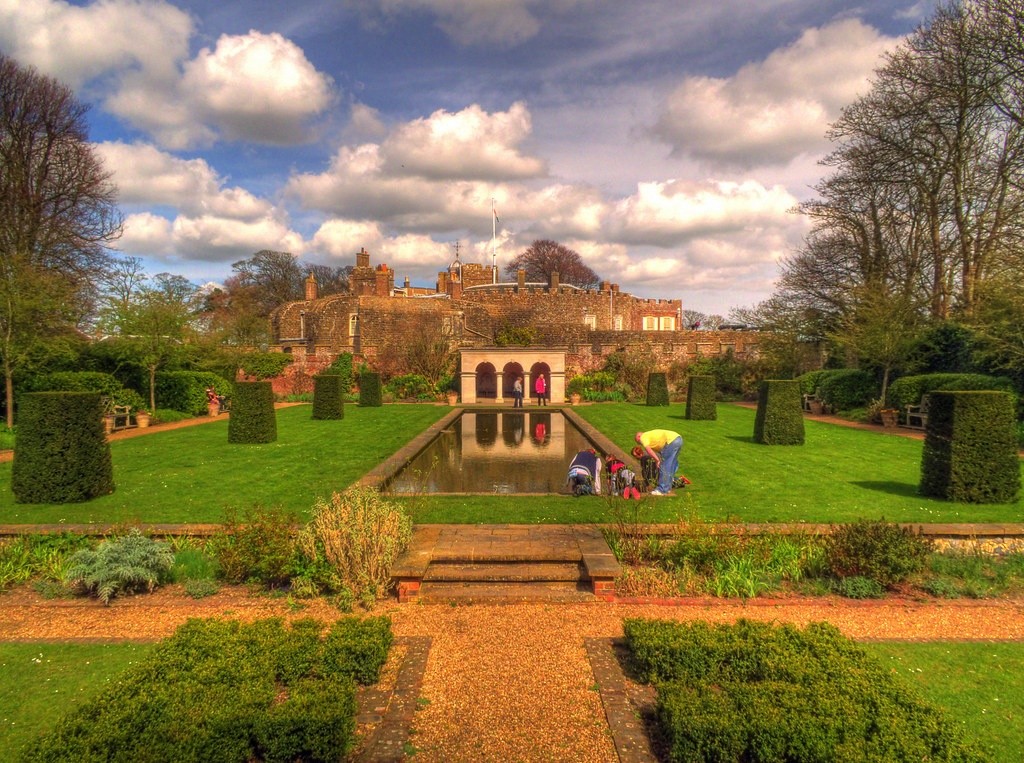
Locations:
[100,393,132,432]
[905,394,933,429]
[804,386,834,415]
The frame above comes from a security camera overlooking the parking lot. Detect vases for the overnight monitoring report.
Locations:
[206,404,220,417]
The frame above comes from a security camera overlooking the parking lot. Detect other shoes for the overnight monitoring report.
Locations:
[623,487,629,500]
[679,475,691,485]
[631,488,641,500]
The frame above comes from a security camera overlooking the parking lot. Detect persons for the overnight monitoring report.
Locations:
[513,377,524,408]
[205,382,232,413]
[565,447,603,498]
[535,374,548,406]
[631,446,692,491]
[635,428,683,495]
[604,454,640,500]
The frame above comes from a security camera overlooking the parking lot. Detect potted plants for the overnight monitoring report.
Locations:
[808,387,824,415]
[879,387,901,428]
[133,397,152,427]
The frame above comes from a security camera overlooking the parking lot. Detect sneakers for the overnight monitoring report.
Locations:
[650,490,663,495]
